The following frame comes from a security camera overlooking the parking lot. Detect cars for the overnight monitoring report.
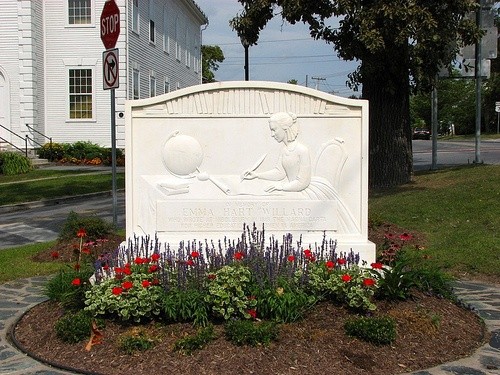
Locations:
[412,126,431,140]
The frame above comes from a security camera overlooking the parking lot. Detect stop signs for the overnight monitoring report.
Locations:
[99,0,120,49]
